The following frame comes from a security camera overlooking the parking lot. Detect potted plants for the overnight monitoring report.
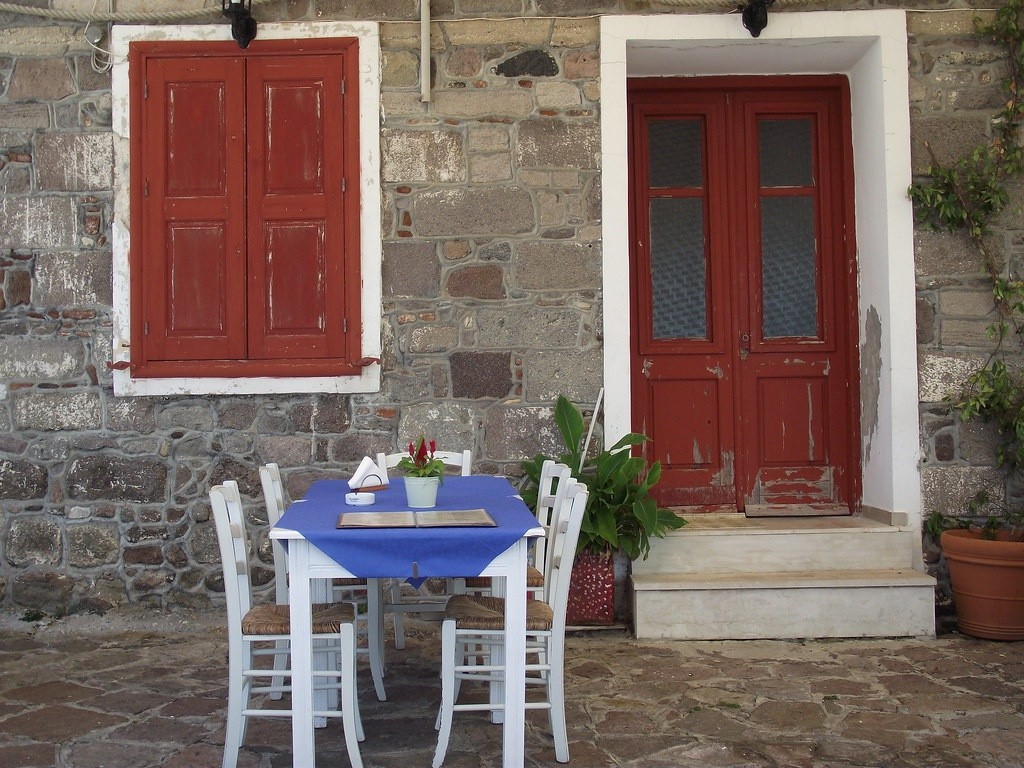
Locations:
[402,471,440,508]
[940,523,1023,640]
[522,396,685,627]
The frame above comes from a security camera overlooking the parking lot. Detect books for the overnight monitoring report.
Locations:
[335,508,498,529]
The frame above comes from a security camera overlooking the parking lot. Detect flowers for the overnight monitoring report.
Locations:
[397,434,446,479]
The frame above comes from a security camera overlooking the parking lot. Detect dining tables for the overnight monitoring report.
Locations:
[269,474,549,766]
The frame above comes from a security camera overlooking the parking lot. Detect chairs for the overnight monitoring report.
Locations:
[428,480,591,768]
[260,457,393,705]
[379,443,478,650]
[428,456,573,730]
[208,478,367,768]
